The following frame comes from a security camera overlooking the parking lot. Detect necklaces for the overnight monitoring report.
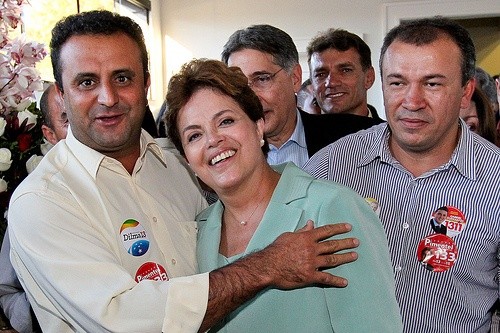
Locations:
[220,174,277,226]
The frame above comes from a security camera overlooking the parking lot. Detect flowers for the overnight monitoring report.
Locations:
[0,0,54,233]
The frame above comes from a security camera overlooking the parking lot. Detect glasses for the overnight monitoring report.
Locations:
[247,62,286,91]
[465,124,483,135]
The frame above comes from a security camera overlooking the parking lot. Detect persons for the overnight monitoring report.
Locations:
[418,247,434,271]
[460,67,500,148]
[306,28,379,118]
[295,78,326,114]
[301,17,500,333]
[221,24,387,168]
[370,202,378,211]
[430,206,448,236]
[163,57,402,333]
[0,83,69,333]
[7,9,360,333]
